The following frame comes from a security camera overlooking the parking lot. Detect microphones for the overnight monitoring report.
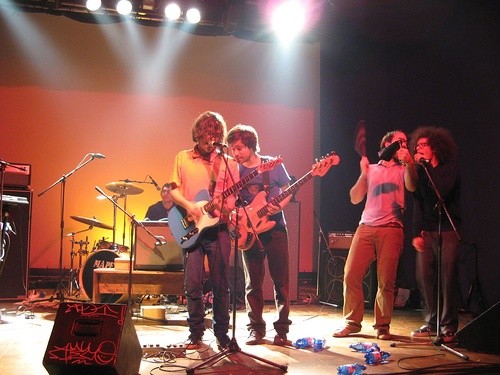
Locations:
[420,158,429,163]
[154,242,166,246]
[90,153,106,158]
[209,141,228,148]
[3,204,11,217]
[149,176,161,191]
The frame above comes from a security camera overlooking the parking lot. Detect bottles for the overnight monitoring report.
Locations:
[292,338,326,352]
[336,362,367,375]
[349,341,392,364]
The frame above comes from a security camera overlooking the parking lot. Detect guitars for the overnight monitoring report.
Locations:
[168,154,283,250]
[226,151,341,251]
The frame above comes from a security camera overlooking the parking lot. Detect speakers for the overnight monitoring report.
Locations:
[41,300,144,375]
[134,221,185,271]
[321,249,378,309]
[454,301,500,357]
[0,189,33,301]
[262,201,301,302]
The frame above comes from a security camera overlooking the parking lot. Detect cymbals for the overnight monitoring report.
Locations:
[105,182,144,195]
[70,215,113,230]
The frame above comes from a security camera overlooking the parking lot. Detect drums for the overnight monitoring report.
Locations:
[79,248,144,304]
[97,240,129,253]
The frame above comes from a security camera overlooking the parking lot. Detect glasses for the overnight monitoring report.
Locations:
[413,142,429,150]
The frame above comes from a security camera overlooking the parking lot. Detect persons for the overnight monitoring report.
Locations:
[333,128,418,340]
[170,111,240,352]
[410,128,466,342]
[226,123,292,345]
[144,183,177,220]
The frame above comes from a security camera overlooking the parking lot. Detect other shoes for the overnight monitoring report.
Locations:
[376,328,391,340]
[440,326,457,337]
[215,335,230,348]
[245,330,261,345]
[411,324,441,336]
[273,334,288,346]
[188,331,204,342]
[332,322,361,338]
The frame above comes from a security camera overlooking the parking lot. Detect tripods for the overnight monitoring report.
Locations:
[290,211,340,309]
[185,146,288,375]
[390,162,471,360]
[49,223,95,302]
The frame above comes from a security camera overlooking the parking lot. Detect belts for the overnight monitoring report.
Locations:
[379,222,402,228]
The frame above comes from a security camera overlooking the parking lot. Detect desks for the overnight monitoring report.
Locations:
[92,268,186,304]
